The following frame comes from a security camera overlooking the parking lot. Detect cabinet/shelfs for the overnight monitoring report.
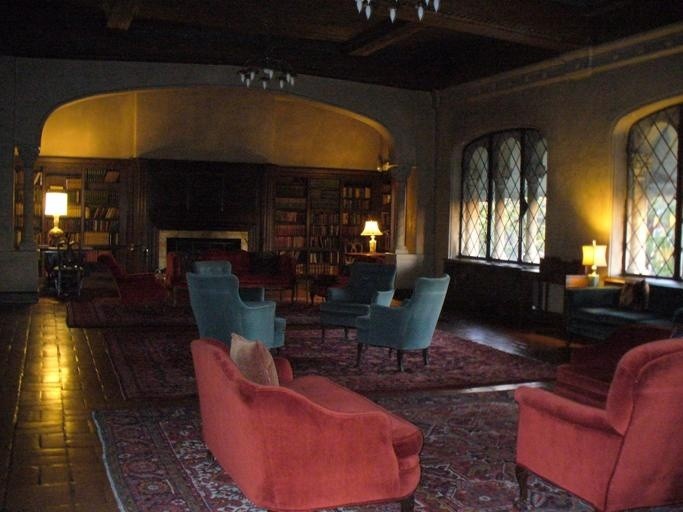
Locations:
[13,156,130,252]
[266,166,392,289]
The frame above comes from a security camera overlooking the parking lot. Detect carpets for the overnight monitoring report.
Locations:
[91,385,594,510]
[65,299,403,328]
[97,325,557,400]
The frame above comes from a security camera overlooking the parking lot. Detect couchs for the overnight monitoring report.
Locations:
[167,250,297,308]
[564,284,683,351]
[186,336,422,512]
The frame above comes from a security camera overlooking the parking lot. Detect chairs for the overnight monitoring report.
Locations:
[183,260,286,349]
[514,325,682,512]
[309,256,450,373]
[97,251,167,312]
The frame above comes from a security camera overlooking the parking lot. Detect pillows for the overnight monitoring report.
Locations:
[229,331,281,388]
[618,276,649,311]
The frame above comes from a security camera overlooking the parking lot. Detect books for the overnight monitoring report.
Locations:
[261,175,392,276]
[14,164,121,276]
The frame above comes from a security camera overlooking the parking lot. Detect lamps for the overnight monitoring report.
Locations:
[580,240,607,288]
[238,53,296,91]
[43,190,67,248]
[359,220,384,253]
